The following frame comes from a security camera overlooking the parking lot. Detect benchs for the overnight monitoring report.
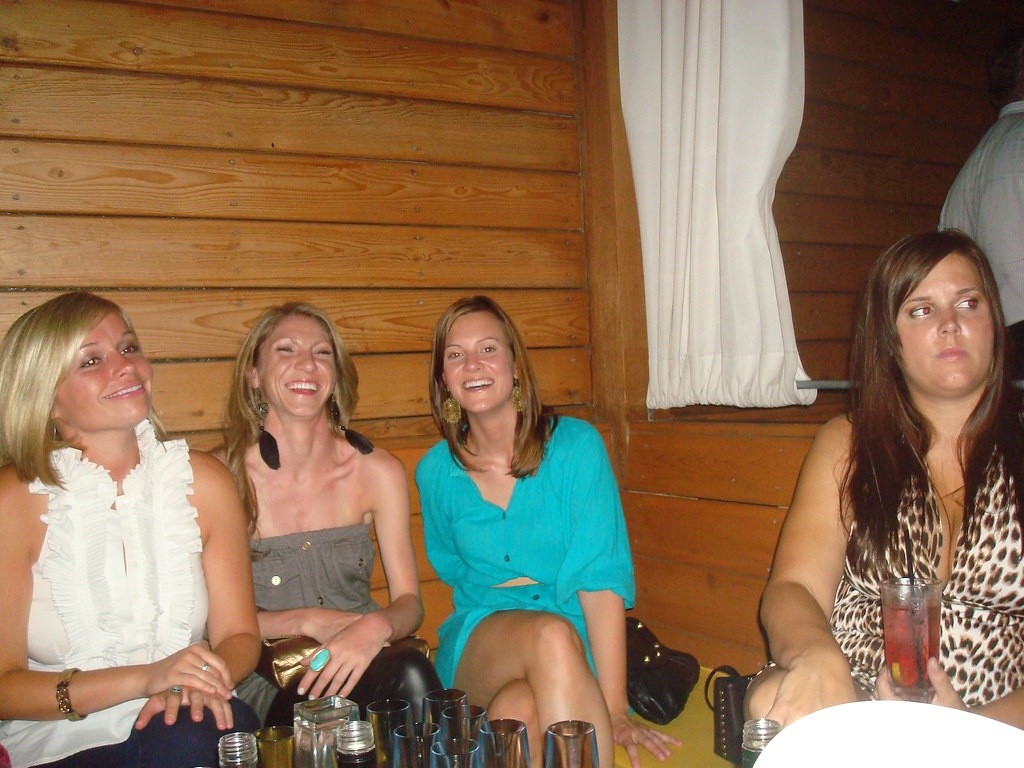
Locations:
[157,406,846,767]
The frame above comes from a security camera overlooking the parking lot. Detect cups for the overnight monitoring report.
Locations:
[440,704,488,741]
[366,699,410,768]
[256,726,294,768]
[479,718,530,768]
[430,738,479,768]
[422,688,469,726]
[881,578,942,703]
[394,722,440,768]
[544,719,599,768]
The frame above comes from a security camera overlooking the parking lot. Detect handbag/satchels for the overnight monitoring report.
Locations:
[625,615,701,725]
[262,629,431,693]
[705,661,755,764]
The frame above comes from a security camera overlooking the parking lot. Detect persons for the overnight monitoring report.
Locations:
[939,25,1024,393]
[0,293,261,768]
[415,296,682,768]
[743,230,1024,733]
[206,302,444,768]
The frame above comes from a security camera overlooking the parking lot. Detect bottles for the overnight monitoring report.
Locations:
[741,718,780,768]
[337,721,377,768]
[218,732,258,768]
[294,695,360,768]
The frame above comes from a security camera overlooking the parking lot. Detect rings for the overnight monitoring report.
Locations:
[172,686,183,693]
[309,648,330,672]
[203,663,210,671]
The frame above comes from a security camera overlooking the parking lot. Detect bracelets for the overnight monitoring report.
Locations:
[55,668,88,721]
